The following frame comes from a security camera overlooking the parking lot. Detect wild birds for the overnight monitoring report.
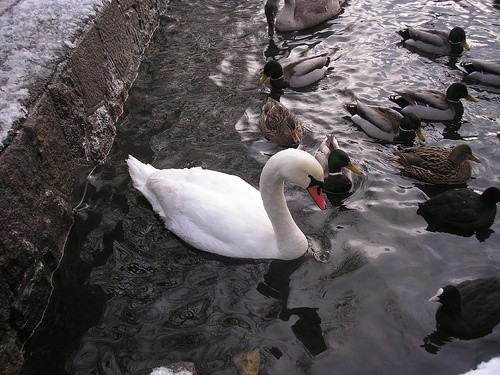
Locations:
[428,276,500,339]
[417,186,500,228]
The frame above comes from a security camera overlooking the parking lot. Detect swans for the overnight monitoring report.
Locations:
[125,147,327,262]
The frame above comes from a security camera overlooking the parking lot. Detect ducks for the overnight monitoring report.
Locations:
[258,53,335,89]
[342,82,478,146]
[257,96,304,147]
[394,24,500,87]
[296,132,363,194]
[392,143,482,185]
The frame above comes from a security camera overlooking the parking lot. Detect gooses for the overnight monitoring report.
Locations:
[264,0,350,38]
